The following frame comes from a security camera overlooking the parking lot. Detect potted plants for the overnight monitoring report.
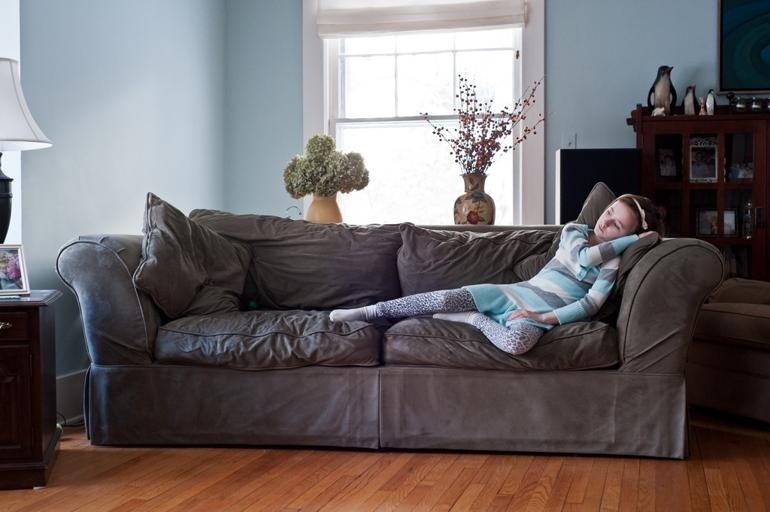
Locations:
[413,74,551,226]
[284,136,369,224]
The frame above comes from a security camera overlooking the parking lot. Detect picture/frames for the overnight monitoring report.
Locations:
[0,244,32,297]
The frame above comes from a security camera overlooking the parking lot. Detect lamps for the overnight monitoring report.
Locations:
[0,58,51,240]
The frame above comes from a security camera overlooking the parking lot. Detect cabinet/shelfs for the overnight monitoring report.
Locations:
[0,290,65,490]
[627,101,770,284]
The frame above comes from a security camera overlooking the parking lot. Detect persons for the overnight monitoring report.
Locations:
[0,250,23,289]
[329,194,666,356]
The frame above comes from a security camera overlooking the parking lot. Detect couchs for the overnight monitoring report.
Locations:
[49,203,729,464]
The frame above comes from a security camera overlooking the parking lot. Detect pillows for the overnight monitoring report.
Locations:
[132,190,249,316]
[513,178,658,316]
